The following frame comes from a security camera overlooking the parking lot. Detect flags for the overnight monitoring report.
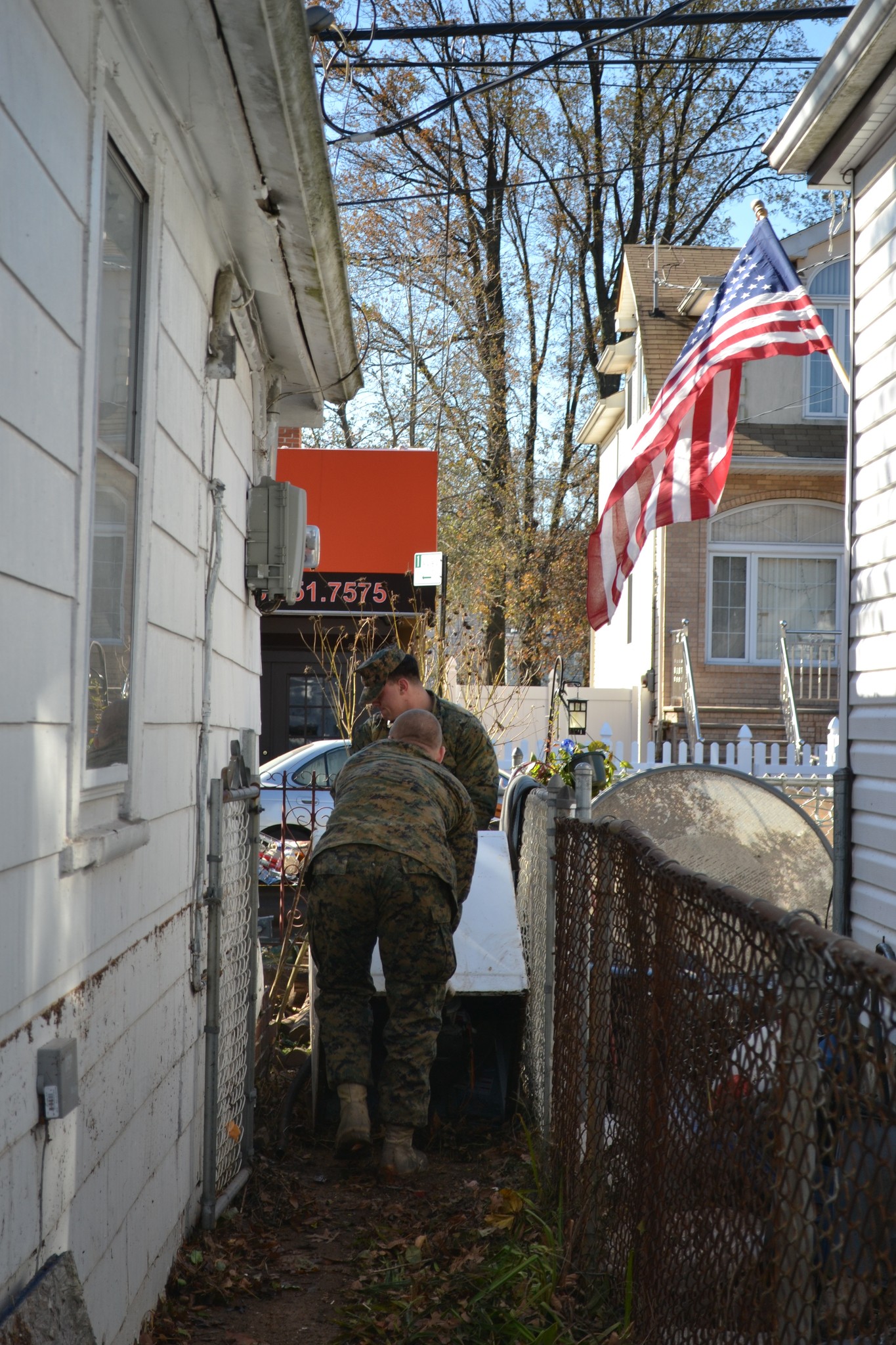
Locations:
[587,217,834,631]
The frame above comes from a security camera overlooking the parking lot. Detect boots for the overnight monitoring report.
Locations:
[333,1081,371,1158]
[378,1123,430,1175]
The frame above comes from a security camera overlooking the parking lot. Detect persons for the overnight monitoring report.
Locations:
[303,708,479,1174]
[349,643,500,831]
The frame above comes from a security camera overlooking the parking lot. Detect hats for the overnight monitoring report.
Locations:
[353,643,406,705]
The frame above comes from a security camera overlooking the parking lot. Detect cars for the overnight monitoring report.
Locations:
[257,738,513,842]
